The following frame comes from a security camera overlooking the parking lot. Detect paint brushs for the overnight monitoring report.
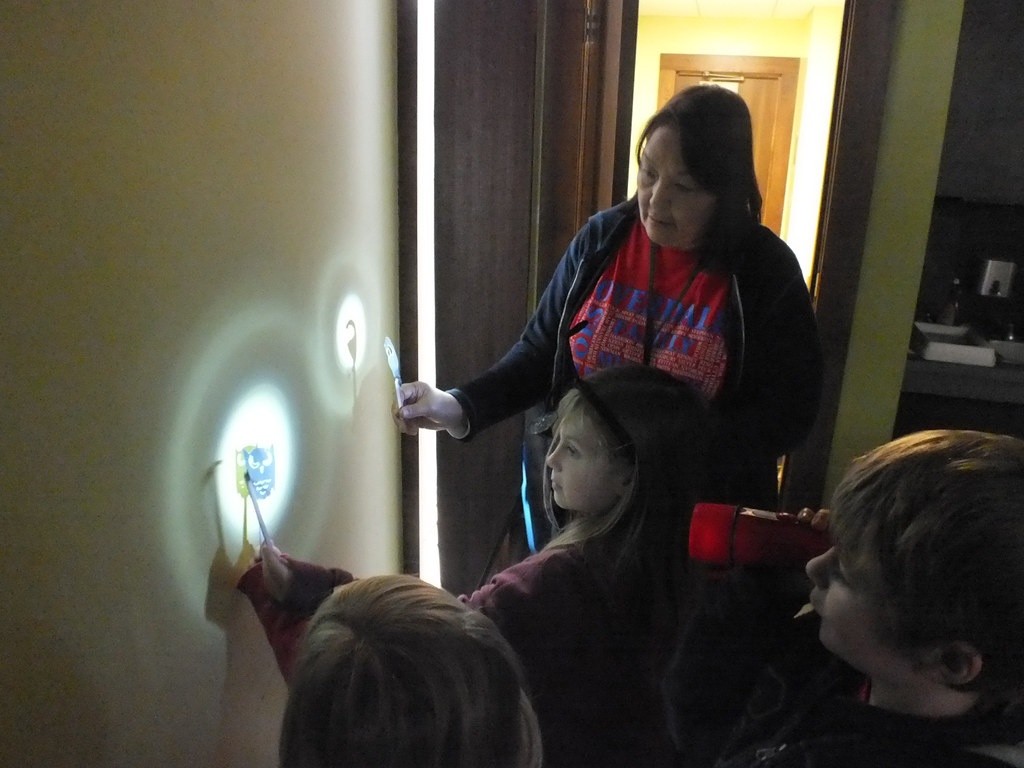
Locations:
[244,472,274,548]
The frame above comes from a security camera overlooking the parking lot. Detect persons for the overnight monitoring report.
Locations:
[273,366,755,768]
[244,539,543,768]
[712,427,1024,768]
[392,85,824,512]
